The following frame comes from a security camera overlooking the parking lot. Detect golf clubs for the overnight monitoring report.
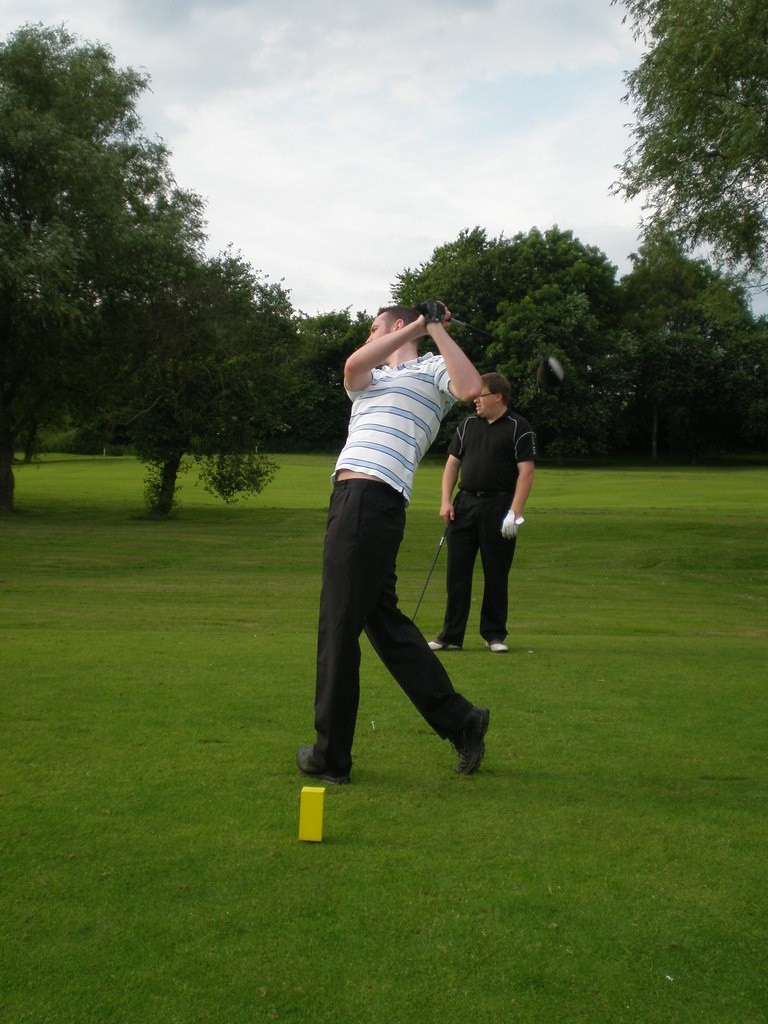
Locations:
[411,515,455,622]
[414,298,568,382]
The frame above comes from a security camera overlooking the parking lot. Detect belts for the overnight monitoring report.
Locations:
[459,490,513,499]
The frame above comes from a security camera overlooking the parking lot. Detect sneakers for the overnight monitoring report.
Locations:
[448,706,490,772]
[296,746,351,786]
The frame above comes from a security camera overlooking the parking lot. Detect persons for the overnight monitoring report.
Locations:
[297,298,492,784]
[427,373,537,654]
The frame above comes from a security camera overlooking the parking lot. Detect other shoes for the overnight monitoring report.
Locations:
[484,639,508,651]
[428,640,462,651]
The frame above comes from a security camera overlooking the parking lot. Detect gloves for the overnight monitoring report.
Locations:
[501,510,524,539]
[420,300,445,329]
